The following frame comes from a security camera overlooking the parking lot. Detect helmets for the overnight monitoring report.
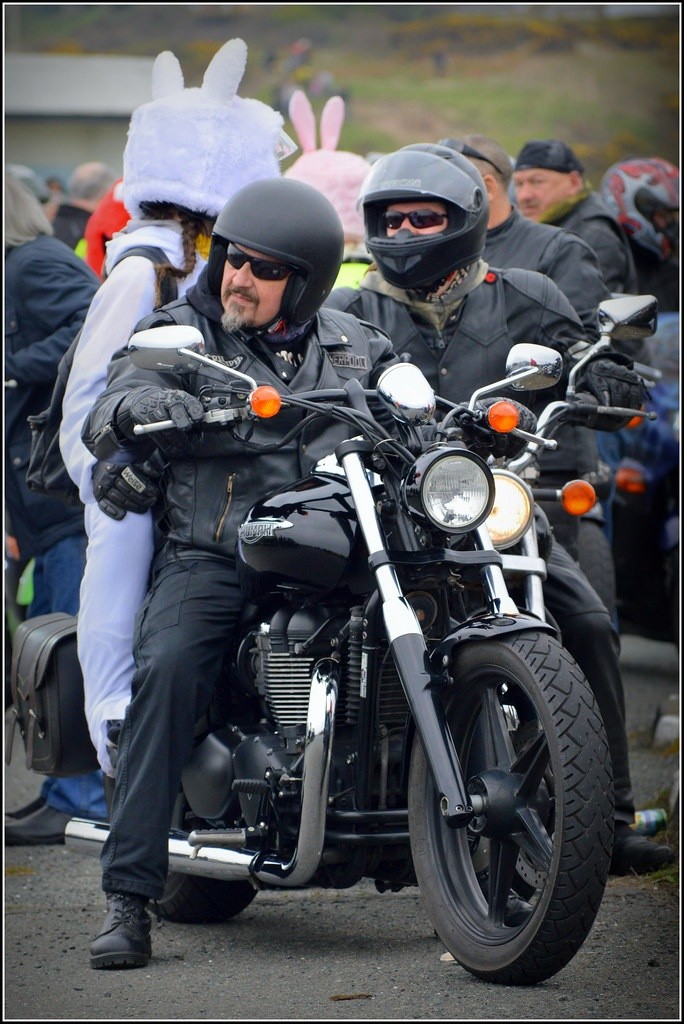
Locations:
[363,142,490,290]
[208,177,345,327]
[600,156,678,260]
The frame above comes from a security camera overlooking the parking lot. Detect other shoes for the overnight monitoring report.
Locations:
[610,827,673,874]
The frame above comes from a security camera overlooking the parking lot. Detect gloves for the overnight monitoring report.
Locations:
[574,358,643,424]
[116,385,204,453]
[93,459,160,521]
[458,395,536,459]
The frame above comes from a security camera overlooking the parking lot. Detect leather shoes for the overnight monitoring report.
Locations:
[88,889,152,967]
[481,875,536,923]
[4,791,79,845]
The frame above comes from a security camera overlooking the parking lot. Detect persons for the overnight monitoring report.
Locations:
[5,166,110,845]
[51,162,116,249]
[602,158,679,316]
[324,142,673,876]
[440,137,614,476]
[60,38,284,782]
[82,178,536,971]
[514,141,635,537]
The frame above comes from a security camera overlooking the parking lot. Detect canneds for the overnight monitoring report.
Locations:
[629,807,669,836]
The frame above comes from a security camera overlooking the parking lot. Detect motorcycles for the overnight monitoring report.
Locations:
[6,298,659,987]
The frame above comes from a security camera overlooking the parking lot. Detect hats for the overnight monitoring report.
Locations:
[515,141,583,176]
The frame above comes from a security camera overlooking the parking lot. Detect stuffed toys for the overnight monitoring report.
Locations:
[84,179,132,281]
[285,91,373,292]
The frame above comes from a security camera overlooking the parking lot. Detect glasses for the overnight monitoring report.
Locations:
[439,137,503,174]
[225,240,293,282]
[381,211,449,228]
[659,208,678,240]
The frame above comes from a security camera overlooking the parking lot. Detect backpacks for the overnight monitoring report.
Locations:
[25,244,178,504]
